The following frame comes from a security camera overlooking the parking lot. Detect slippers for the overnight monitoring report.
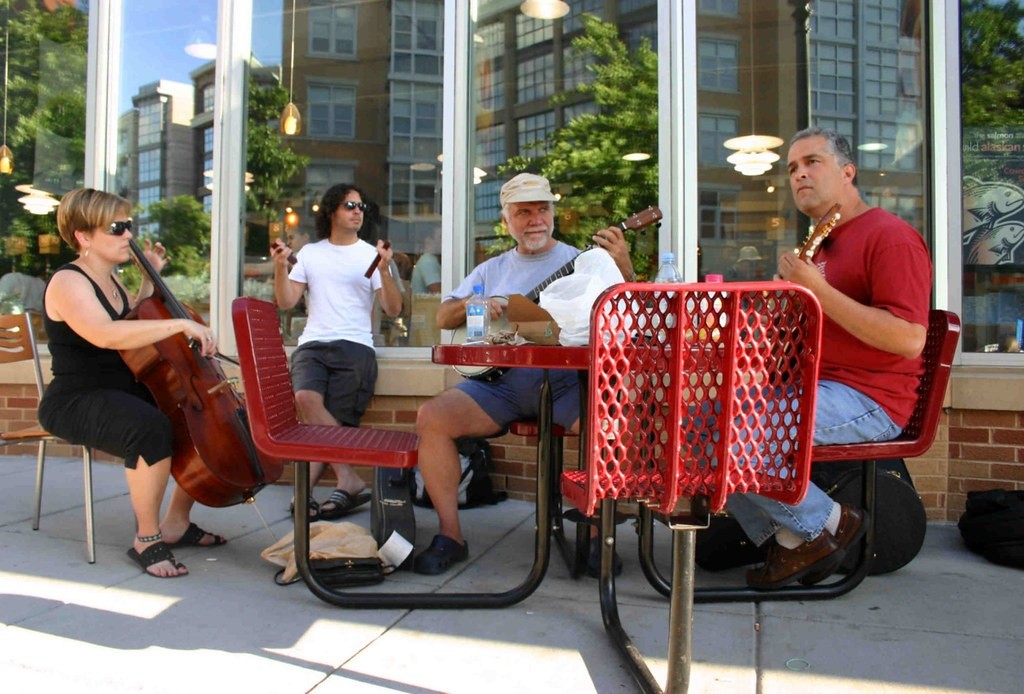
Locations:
[290,496,320,522]
[414,534,469,575]
[127,541,189,578]
[320,489,372,519]
[165,523,228,549]
[590,537,622,579]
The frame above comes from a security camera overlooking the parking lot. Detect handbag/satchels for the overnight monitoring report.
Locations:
[274,557,398,588]
[261,519,378,582]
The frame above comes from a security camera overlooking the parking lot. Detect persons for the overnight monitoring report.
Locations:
[415,170,638,578]
[683,127,933,593]
[37,188,227,577]
[359,200,406,336]
[269,183,403,521]
[410,223,442,293]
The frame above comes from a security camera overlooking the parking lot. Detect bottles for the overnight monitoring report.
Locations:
[655,253,683,347]
[699,274,728,348]
[465,284,491,341]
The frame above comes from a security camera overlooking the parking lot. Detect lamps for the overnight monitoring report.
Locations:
[0,0,895,223]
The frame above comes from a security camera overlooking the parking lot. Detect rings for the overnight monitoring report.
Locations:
[207,337,212,341]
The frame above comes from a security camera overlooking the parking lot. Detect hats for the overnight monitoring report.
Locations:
[499,173,558,208]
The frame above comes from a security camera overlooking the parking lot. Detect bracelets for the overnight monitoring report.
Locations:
[627,271,636,281]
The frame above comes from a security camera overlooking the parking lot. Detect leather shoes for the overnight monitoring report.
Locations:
[796,503,870,585]
[747,529,846,590]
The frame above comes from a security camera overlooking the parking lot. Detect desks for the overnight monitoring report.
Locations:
[432,343,767,610]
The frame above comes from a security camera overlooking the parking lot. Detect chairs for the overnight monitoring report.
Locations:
[0,311,96,564]
[233,295,960,694]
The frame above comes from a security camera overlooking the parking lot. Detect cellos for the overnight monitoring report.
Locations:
[118,236,279,545]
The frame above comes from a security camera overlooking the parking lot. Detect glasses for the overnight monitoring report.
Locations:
[339,201,367,210]
[104,220,131,236]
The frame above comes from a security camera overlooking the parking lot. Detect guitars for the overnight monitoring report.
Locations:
[793,202,842,262]
[451,204,663,381]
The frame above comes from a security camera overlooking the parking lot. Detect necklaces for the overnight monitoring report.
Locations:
[81,257,119,300]
